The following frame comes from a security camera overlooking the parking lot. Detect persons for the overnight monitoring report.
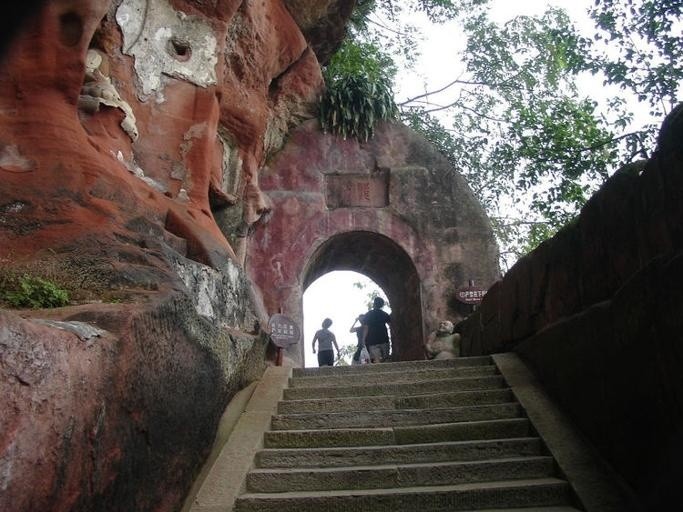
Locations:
[312,319,340,367]
[350,297,391,365]
[425,321,461,359]
